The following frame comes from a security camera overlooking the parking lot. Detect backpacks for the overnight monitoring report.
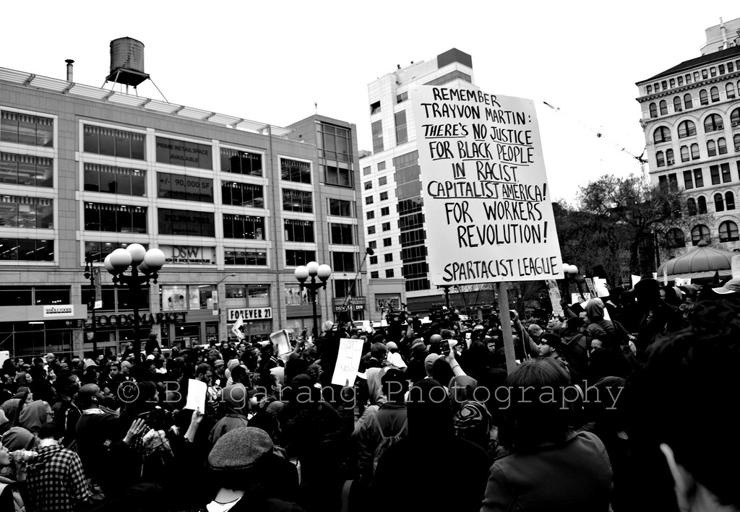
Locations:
[370,411,407,499]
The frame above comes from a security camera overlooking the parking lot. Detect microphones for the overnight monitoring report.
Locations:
[366,247,374,255]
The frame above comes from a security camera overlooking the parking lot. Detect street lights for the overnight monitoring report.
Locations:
[105,244,166,368]
[85,253,99,355]
[296,262,330,343]
[561,260,578,304]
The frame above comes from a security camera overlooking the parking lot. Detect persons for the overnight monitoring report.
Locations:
[0,272,740,512]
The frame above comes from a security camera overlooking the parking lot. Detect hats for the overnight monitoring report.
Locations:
[381,369,407,386]
[425,353,450,379]
[540,334,561,348]
[207,426,274,472]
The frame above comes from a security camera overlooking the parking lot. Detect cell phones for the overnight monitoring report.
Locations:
[440,340,450,356]
